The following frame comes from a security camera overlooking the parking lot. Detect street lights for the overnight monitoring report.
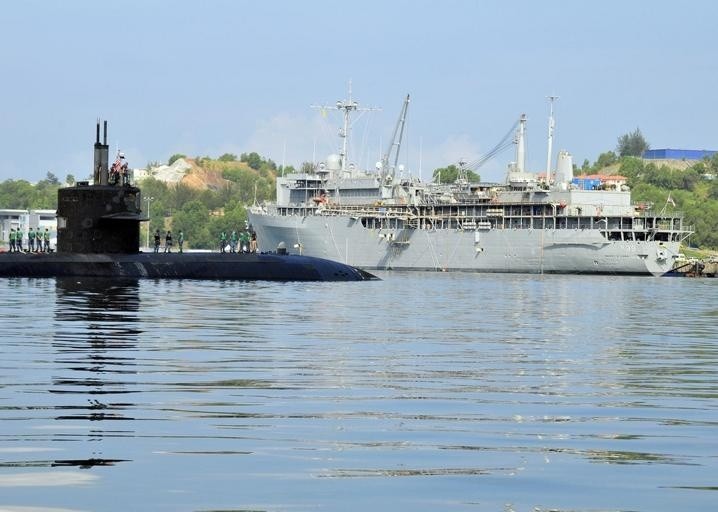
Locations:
[142,197,154,250]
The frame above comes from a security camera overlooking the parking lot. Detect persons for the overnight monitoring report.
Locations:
[8,228,16,252]
[229,229,239,253]
[250,230,257,255]
[15,226,24,252]
[153,229,161,253]
[27,227,37,253]
[175,229,185,253]
[243,228,252,253]
[120,161,129,184]
[237,229,244,253]
[35,227,43,253]
[42,228,51,252]
[164,230,172,253]
[109,163,120,185]
[219,228,229,254]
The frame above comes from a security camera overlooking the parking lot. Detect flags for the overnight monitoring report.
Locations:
[112,151,122,174]
[668,195,676,207]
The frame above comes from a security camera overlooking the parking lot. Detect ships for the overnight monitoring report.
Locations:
[244,81,696,277]
[0,120,382,281]
